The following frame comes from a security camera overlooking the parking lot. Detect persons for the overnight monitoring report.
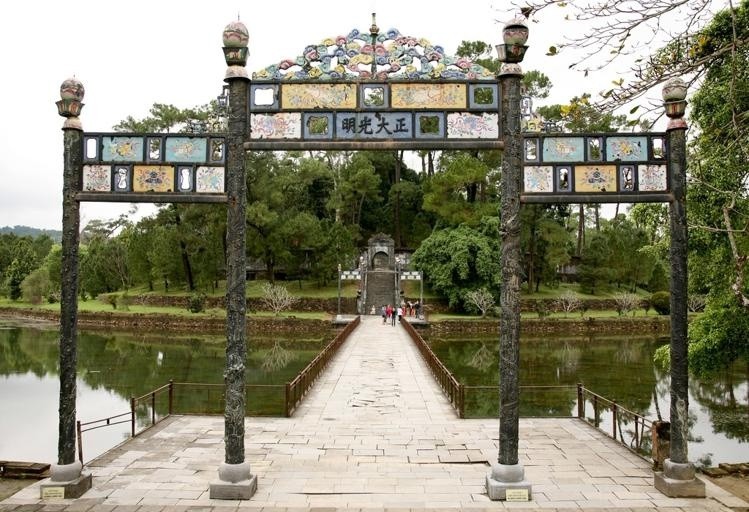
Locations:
[380,299,420,327]
[370,304,376,315]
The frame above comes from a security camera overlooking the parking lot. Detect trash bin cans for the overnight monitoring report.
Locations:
[652,421,670,470]
